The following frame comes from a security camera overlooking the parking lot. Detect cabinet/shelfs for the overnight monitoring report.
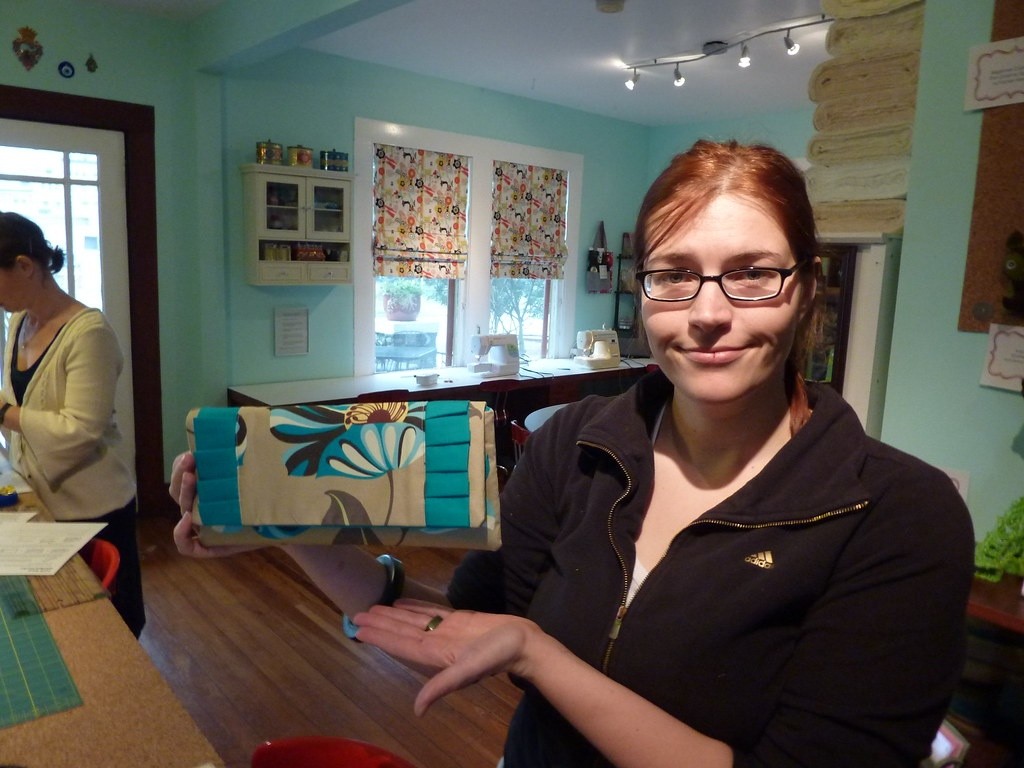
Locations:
[240,162,354,286]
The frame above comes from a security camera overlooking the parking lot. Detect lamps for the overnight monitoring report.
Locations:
[739,42,751,68]
[624,68,640,90]
[674,62,685,86]
[784,28,800,56]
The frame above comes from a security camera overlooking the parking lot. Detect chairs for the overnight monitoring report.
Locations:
[252,738,416,768]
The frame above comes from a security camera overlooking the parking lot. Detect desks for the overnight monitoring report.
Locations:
[0,493,227,768]
[228,359,665,579]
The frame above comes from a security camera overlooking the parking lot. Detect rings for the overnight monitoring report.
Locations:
[423,615,443,633]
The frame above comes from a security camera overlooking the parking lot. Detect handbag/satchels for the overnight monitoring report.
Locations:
[184,399,503,551]
[586,221,614,294]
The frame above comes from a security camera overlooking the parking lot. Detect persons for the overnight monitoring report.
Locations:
[0,211,148,647]
[164,136,976,768]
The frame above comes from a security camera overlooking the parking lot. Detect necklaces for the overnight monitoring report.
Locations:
[21,304,58,350]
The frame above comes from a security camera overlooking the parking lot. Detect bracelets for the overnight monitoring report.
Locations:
[0,402,14,425]
[342,554,407,644]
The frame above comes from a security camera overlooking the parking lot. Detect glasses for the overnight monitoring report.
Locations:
[635,258,808,302]
